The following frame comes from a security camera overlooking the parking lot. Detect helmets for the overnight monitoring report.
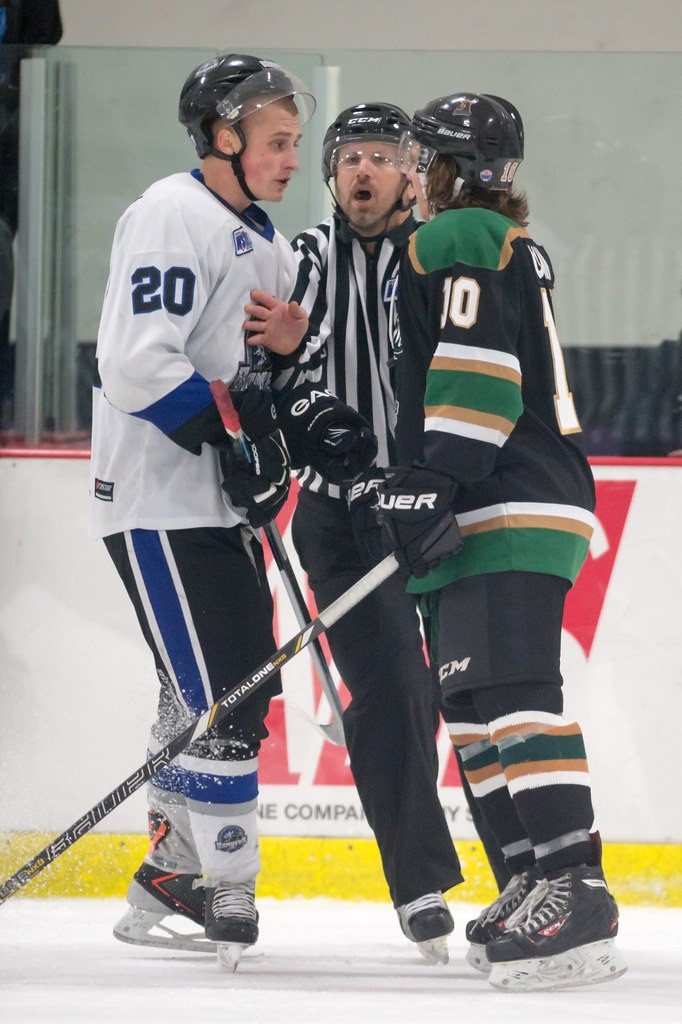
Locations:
[321,101,412,182]
[178,53,293,159]
[408,93,524,190]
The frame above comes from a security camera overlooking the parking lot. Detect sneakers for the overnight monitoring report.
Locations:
[396,888,456,964]
[113,856,259,953]
[466,831,627,992]
[204,871,259,971]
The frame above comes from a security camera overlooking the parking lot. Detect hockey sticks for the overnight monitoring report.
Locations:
[0,550,402,907]
[211,379,350,750]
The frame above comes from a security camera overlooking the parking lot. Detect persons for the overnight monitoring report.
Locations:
[348,91,637,992]
[93,55,380,974]
[243,100,466,965]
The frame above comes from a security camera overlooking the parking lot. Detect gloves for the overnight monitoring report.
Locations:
[221,382,291,528]
[348,467,464,578]
[278,382,378,484]
